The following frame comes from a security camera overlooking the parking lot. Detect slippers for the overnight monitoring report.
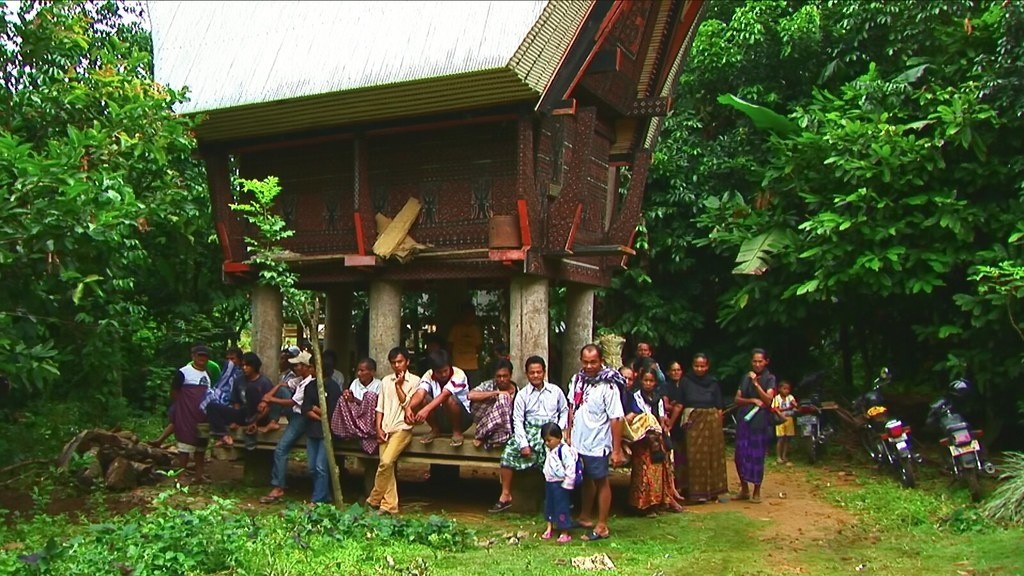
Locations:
[488,501,513,513]
[581,531,610,541]
[450,437,462,447]
[571,519,593,528]
[260,495,278,503]
[419,433,439,443]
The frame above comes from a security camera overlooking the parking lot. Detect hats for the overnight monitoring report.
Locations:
[288,346,300,356]
[191,346,208,355]
[287,350,312,366]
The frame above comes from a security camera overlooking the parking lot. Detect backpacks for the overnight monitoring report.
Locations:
[558,444,583,485]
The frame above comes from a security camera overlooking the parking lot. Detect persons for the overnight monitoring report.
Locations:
[405,348,474,447]
[732,348,774,503]
[770,380,798,464]
[337,357,381,439]
[488,355,568,513]
[538,422,577,543]
[566,344,623,541]
[467,358,520,447]
[618,343,724,518]
[364,347,420,516]
[144,340,345,509]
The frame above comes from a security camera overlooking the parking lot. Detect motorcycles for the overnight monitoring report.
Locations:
[795,387,834,465]
[925,394,996,503]
[851,367,922,489]
[721,402,737,445]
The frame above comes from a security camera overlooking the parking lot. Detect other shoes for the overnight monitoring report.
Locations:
[542,531,554,538]
[245,428,256,451]
[748,497,762,503]
[730,494,750,500]
[647,510,659,517]
[557,535,572,542]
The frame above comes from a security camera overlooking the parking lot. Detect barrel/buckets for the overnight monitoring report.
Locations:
[488,216,521,248]
